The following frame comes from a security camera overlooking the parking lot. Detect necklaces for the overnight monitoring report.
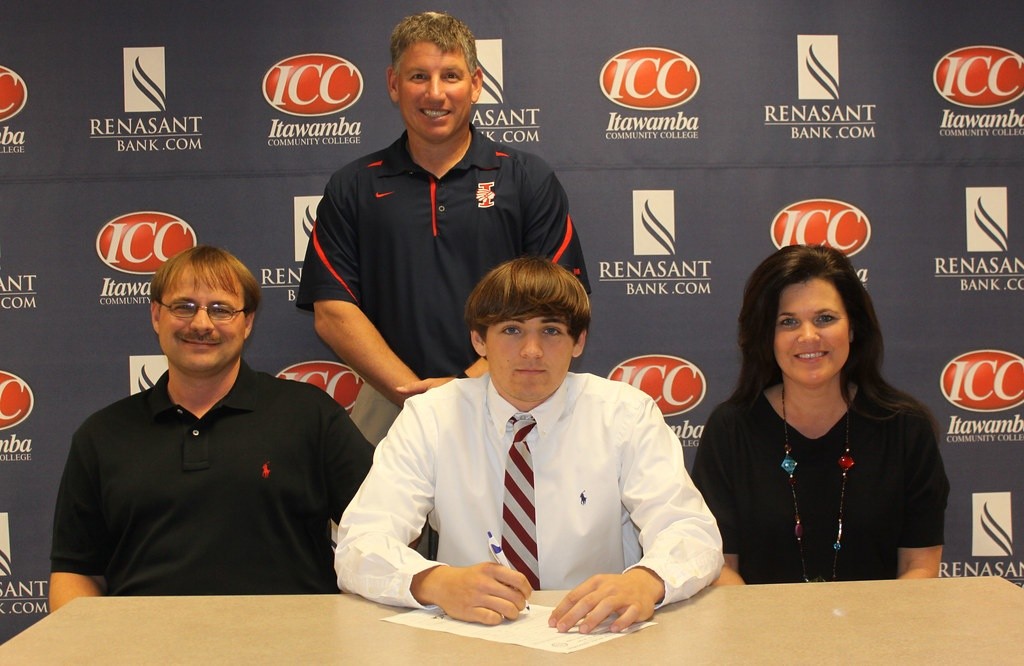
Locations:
[782,380,851,582]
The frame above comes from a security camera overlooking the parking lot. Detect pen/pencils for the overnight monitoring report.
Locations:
[486,531,530,611]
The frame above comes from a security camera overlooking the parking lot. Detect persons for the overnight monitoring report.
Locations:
[295,11,591,446]
[689,242,950,588]
[49,248,374,611]
[336,259,722,635]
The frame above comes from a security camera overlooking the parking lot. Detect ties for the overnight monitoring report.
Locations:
[501,416,541,591]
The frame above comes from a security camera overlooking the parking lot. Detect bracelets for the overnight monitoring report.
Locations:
[455,371,471,380]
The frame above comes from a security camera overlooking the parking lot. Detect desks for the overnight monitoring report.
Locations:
[0,574,1024,666]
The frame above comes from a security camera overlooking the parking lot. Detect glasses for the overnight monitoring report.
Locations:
[160,301,243,320]
